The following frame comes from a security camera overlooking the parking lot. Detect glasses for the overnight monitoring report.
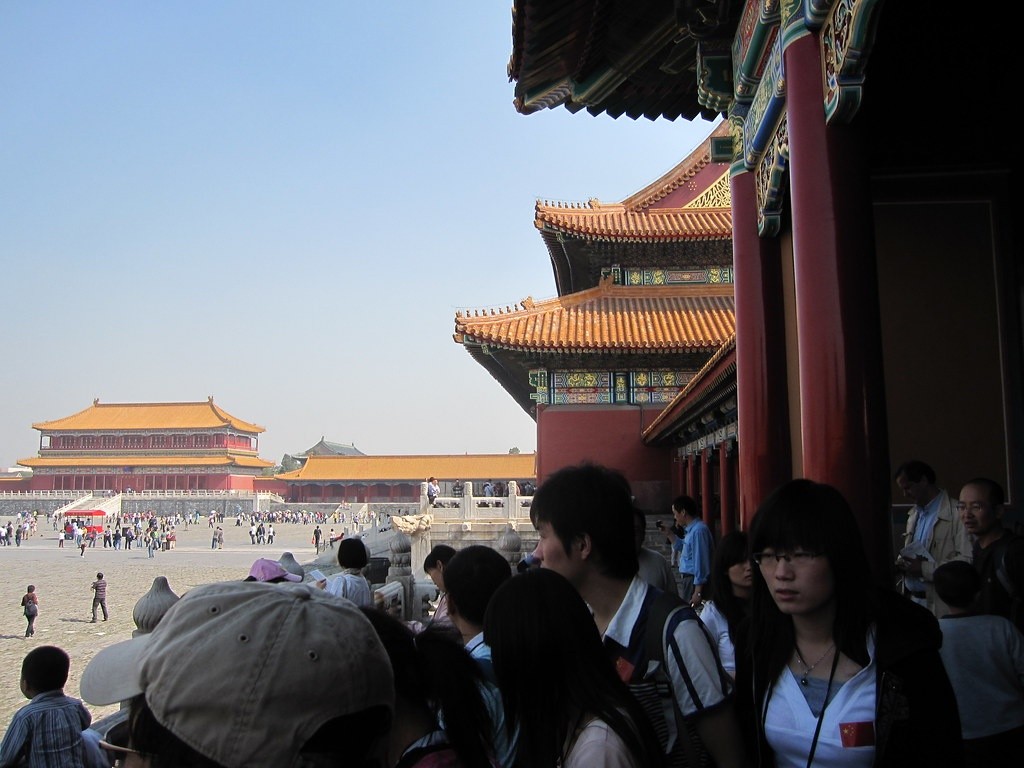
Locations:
[752,551,820,563]
[98,720,153,767]
[955,502,983,513]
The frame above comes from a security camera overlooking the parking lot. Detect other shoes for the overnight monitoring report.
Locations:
[102,619,108,622]
[90,620,98,623]
[80,553,84,558]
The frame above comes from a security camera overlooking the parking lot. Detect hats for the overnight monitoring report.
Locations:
[249,558,302,582]
[77,580,395,767]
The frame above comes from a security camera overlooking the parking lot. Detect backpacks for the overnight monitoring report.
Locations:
[26,594,37,616]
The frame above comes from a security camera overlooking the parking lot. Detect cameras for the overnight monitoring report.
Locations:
[655,519,665,529]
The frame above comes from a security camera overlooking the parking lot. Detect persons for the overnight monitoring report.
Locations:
[0,461,1024,768]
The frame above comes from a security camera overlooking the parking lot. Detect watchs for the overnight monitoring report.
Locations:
[696,592,702,597]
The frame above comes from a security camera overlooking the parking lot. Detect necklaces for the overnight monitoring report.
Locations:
[793,642,837,689]
